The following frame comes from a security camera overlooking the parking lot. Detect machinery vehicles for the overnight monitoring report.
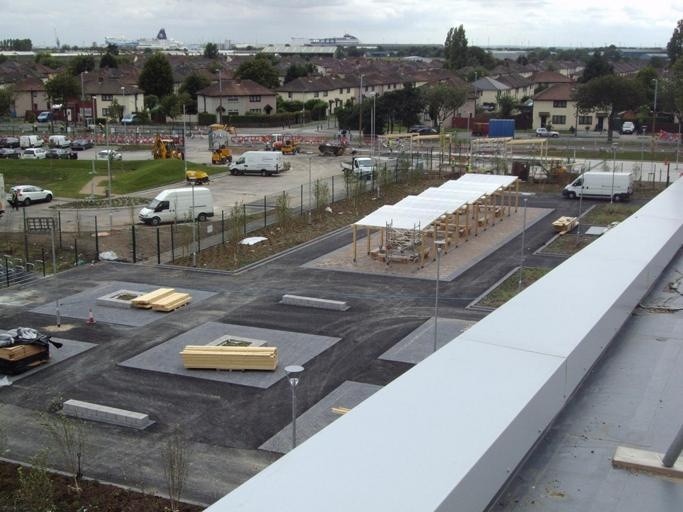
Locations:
[266,130,350,157]
[207,124,237,165]
[151,135,184,162]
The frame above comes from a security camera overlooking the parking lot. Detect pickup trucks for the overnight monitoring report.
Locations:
[340,156,376,180]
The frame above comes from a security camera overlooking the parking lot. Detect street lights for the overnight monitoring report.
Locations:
[431,239,447,351]
[214,68,223,124]
[187,172,198,266]
[673,116,681,168]
[284,364,304,448]
[118,86,126,137]
[576,165,586,247]
[518,191,536,293]
[81,71,89,125]
[91,96,97,175]
[367,91,379,202]
[359,73,366,147]
[650,79,658,135]
[610,141,618,205]
[638,125,647,183]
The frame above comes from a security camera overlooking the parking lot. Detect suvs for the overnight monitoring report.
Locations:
[7,185,52,206]
[536,128,559,138]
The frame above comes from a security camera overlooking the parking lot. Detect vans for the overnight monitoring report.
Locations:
[622,121,634,134]
[138,184,214,226]
[561,171,635,201]
[36,111,52,123]
[121,113,140,125]
[228,150,284,177]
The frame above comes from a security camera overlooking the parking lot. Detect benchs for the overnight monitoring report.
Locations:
[369,204,505,263]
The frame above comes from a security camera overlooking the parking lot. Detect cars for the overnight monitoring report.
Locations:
[0,134,92,160]
[95,150,121,161]
[418,127,439,136]
[408,125,431,133]
